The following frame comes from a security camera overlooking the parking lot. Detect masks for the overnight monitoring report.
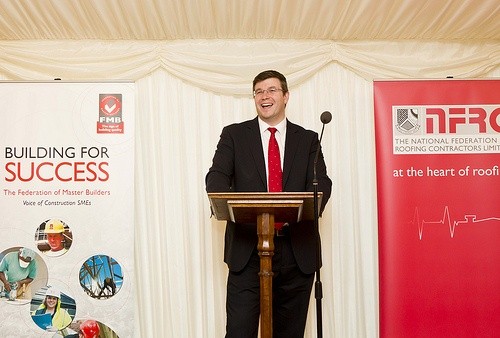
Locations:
[18,258,30,268]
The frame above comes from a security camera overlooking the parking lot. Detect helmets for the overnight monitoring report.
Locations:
[80,320,100,338]
[45,287,61,298]
[44,220,64,234]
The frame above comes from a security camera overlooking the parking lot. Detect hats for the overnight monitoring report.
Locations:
[20,248,34,261]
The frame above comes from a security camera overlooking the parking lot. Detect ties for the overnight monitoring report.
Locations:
[267,128,286,230]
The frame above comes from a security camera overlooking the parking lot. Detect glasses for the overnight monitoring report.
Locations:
[254,88,284,97]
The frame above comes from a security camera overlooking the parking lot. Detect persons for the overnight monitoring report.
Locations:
[42,219,68,257]
[205,70,332,338]
[64,320,101,338]
[99,278,116,297]
[33,287,71,331]
[0,247,37,298]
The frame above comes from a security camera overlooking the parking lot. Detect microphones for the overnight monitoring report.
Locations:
[312,111,331,185]
